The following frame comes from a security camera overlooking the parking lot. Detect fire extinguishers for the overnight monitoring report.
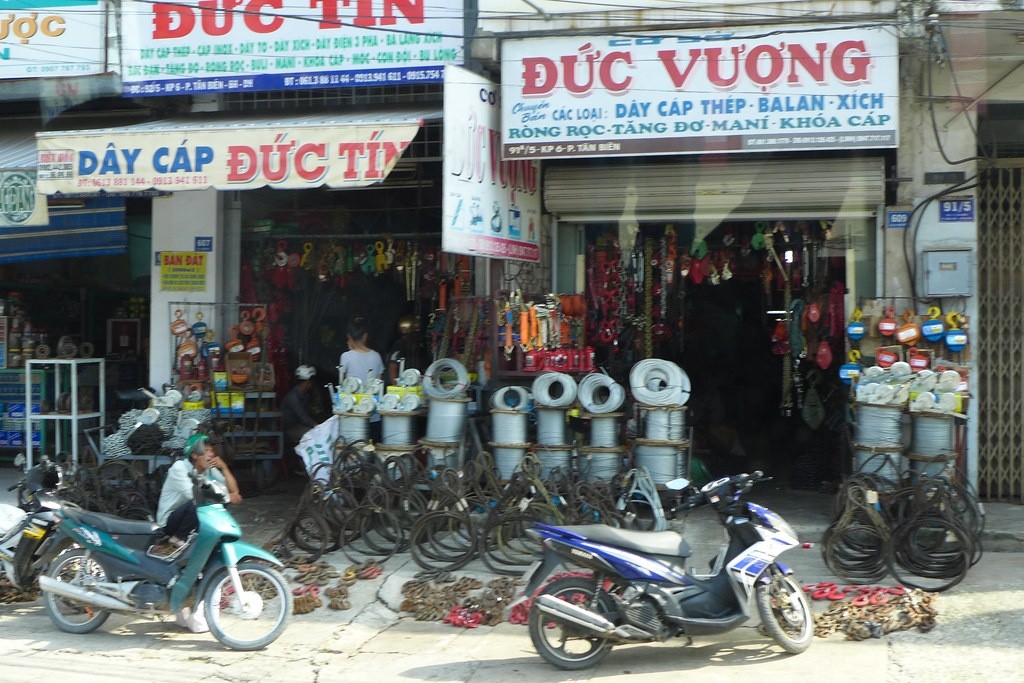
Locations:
[527,343,597,372]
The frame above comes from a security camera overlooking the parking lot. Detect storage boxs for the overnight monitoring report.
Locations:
[0,402,42,447]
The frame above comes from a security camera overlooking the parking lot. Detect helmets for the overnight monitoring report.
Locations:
[295,364,317,381]
[184,434,220,459]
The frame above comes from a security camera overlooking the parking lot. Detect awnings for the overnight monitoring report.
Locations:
[35,105,444,195]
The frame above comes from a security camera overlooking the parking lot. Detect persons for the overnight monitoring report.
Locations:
[338,318,385,390]
[282,365,331,442]
[156,434,242,547]
[389,314,432,386]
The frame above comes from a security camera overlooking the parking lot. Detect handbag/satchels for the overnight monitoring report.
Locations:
[294,415,340,486]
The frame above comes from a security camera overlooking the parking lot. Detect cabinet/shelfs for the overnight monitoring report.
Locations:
[1,369,68,465]
[209,410,284,459]
[23,354,107,476]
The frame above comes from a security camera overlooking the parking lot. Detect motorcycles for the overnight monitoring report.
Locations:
[506,470,813,671]
[0,469,295,650]
